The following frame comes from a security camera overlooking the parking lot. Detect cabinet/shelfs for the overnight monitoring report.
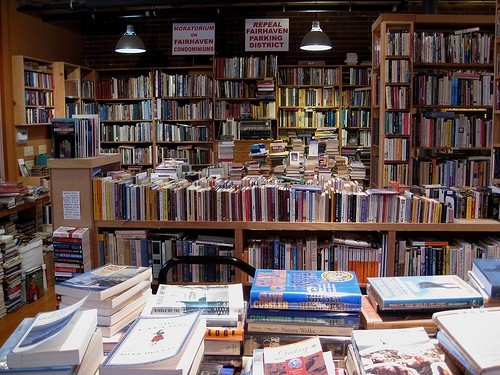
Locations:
[0,14,500,297]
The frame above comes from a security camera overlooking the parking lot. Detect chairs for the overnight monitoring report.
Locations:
[156,256,258,300]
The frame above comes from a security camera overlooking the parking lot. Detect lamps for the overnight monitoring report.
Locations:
[300,20,332,51]
[114,18,146,53]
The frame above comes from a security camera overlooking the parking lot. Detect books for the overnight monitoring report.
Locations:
[0,27,500,375]
